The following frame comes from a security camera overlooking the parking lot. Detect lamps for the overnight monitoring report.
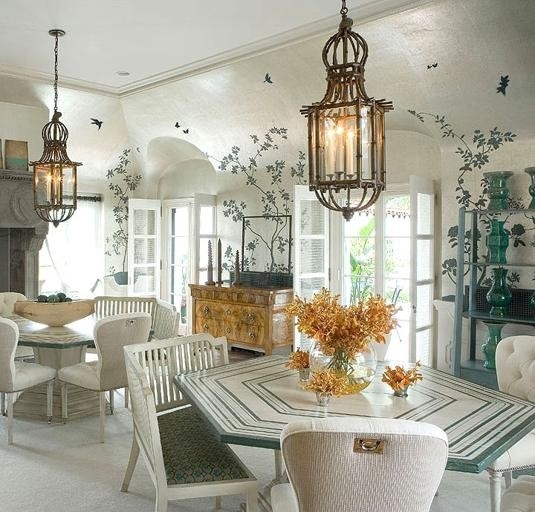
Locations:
[299,0,393,222]
[28,29,82,229]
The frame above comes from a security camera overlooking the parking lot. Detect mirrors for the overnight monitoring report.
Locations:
[242,215,292,273]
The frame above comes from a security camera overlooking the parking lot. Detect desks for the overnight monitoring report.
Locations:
[172,355,535,512]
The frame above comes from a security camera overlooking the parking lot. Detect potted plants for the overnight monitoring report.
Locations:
[103,148,143,286]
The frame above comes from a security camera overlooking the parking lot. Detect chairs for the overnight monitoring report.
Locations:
[486,335,535,511]
[0,291,181,443]
[270,416,448,512]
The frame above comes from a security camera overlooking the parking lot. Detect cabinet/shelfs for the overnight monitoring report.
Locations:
[188,280,296,357]
[453,205,535,392]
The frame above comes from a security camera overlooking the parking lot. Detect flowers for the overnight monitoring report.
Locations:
[285,347,312,371]
[284,287,401,385]
[382,360,423,390]
[305,367,349,398]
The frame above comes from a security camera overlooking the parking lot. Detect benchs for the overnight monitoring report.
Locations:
[120,333,257,511]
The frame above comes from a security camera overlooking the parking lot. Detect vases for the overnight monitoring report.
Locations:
[360,332,392,369]
[480,167,535,370]
[393,387,408,398]
[297,369,310,384]
[308,340,377,395]
[315,390,332,406]
